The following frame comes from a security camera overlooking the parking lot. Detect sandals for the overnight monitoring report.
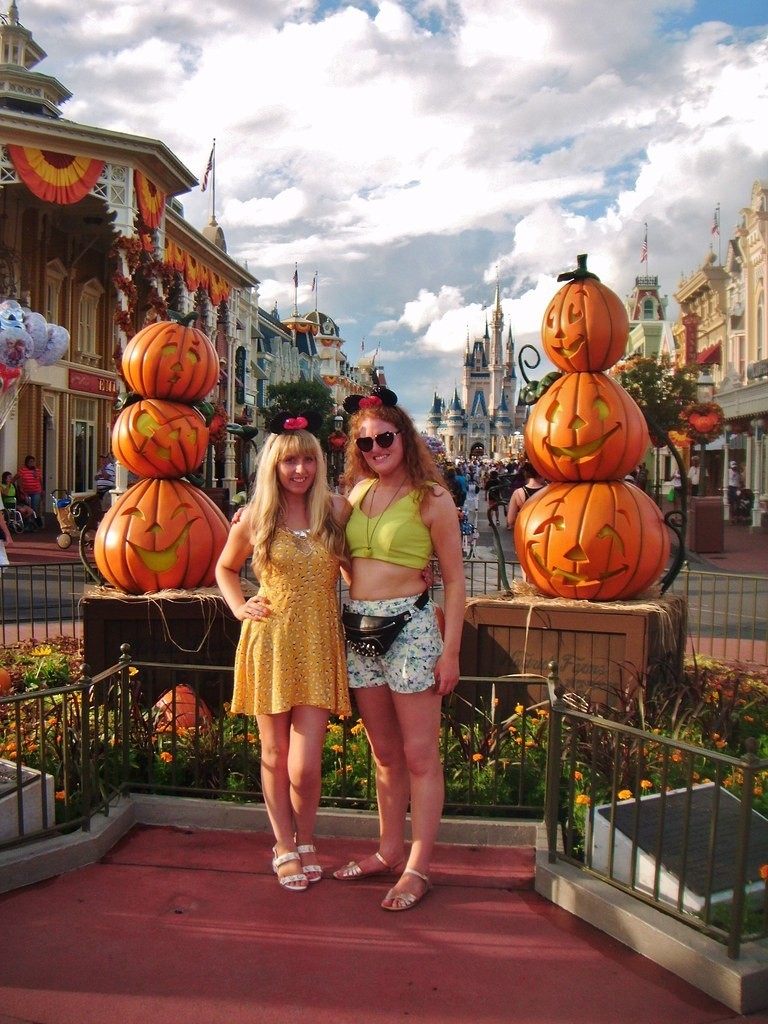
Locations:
[297,844,323,883]
[272,846,308,892]
[381,868,433,911]
[333,852,406,881]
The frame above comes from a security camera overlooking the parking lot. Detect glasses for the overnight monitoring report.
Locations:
[356,429,401,452]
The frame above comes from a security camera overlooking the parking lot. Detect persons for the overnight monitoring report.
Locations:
[229,386,465,909]
[667,467,684,509]
[10,455,44,531]
[626,462,648,493]
[0,471,34,531]
[436,456,550,532]
[215,411,434,890]
[728,462,743,516]
[686,455,710,496]
[93,452,116,499]
[0,493,15,578]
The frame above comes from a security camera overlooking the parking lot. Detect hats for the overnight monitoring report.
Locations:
[729,461,738,468]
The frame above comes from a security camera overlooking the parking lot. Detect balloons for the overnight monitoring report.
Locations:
[0,298,70,393]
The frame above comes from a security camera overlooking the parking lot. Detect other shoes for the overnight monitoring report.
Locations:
[496,520,501,525]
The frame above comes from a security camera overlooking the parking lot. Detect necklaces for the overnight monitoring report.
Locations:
[278,508,311,540]
[363,473,410,557]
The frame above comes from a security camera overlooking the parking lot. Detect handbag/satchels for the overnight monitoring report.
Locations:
[475,486,480,493]
[341,590,429,657]
[15,491,25,501]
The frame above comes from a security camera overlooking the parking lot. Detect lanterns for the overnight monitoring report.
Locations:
[92,478,230,594]
[110,396,209,478]
[521,372,649,484]
[121,318,220,403]
[540,276,630,373]
[514,480,671,603]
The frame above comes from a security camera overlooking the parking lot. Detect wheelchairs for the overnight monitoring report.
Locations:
[3,496,42,534]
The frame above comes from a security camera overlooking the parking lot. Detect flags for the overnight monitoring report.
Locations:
[711,211,720,236]
[201,149,214,191]
[310,277,315,291]
[640,234,648,263]
[293,270,298,287]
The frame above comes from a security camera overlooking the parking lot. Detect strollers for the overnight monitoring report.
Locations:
[50,490,100,551]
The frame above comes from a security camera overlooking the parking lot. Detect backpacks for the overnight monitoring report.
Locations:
[488,486,500,500]
